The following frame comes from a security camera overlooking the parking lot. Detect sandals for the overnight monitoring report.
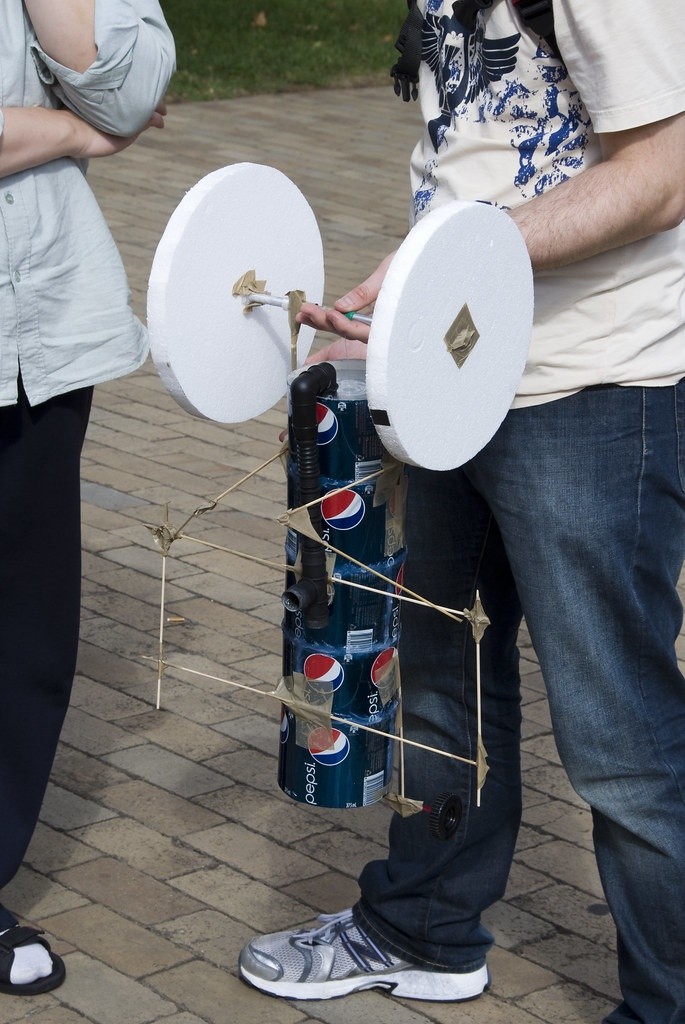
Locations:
[0,909,66,996]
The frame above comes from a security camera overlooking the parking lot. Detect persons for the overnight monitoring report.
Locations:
[0,0,176,997]
[240,0,685,1024]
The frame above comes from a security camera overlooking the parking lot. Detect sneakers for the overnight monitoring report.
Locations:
[238,908,489,1001]
